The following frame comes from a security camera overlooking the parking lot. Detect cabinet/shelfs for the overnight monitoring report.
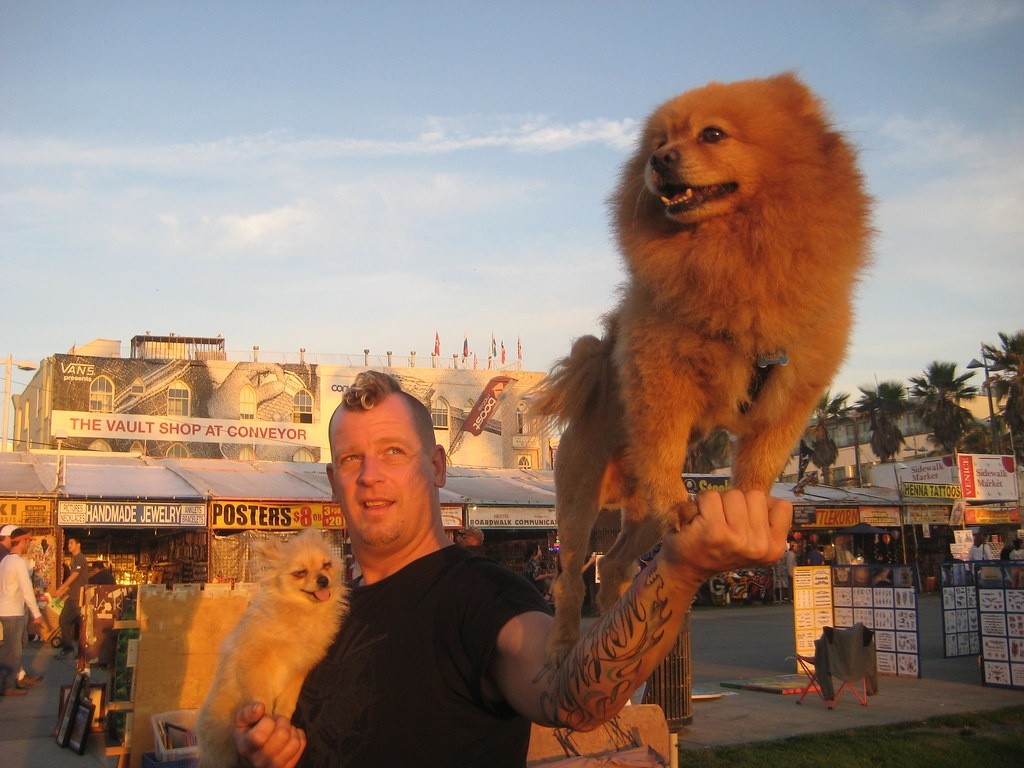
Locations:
[66,530,208,572]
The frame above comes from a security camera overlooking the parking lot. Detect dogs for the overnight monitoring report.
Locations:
[517,66,873,683]
[191,529,350,768]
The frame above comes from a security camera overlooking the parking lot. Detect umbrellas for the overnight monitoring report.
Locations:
[836,522,890,562]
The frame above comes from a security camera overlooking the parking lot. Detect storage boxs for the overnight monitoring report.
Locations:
[142,709,202,768]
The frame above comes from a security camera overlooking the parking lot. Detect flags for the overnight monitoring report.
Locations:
[518,337,522,361]
[491,333,497,357]
[464,334,469,356]
[488,355,493,371]
[501,343,506,363]
[435,332,441,356]
[473,355,478,369]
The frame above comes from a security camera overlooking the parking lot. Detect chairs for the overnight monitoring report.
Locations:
[794,622,878,710]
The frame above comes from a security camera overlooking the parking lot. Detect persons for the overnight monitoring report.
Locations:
[967,532,1024,589]
[777,538,826,605]
[0,523,117,695]
[457,524,596,607]
[229,370,793,768]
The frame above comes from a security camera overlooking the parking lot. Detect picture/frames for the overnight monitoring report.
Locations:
[53,683,106,754]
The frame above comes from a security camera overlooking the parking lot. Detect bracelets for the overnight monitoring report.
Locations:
[545,591,551,596]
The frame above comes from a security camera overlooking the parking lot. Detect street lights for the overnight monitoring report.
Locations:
[966,341,1001,455]
[0,354,37,452]
